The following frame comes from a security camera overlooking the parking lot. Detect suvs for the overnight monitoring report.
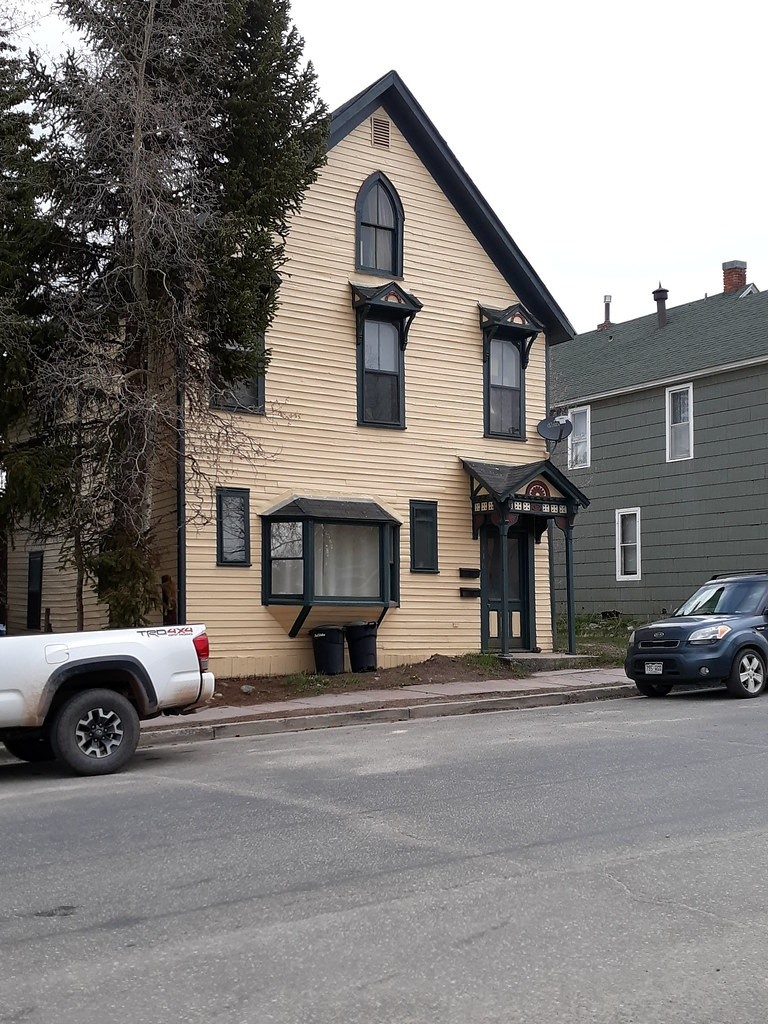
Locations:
[623,568,768,699]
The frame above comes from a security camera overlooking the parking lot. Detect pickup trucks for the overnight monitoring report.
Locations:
[0,623,215,778]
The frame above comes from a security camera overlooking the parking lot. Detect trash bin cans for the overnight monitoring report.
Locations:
[309,624,344,675]
[344,620,378,673]
